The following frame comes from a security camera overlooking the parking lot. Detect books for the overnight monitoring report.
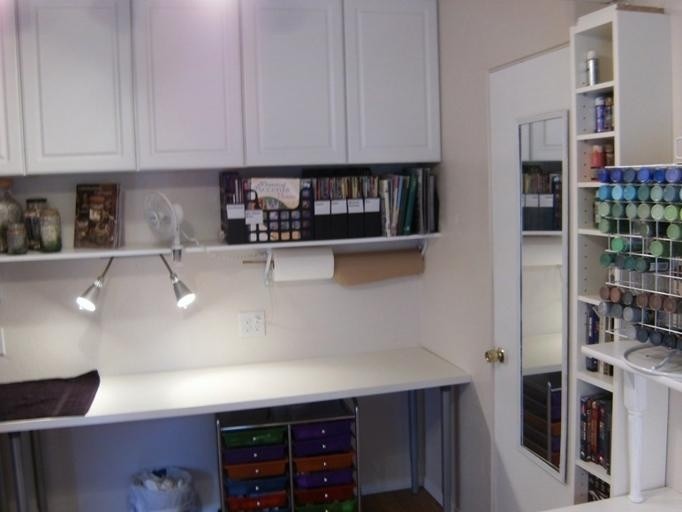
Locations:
[580,390,613,474]
[228,164,439,237]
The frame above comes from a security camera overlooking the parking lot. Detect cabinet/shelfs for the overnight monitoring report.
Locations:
[0,0,440,177]
[220,398,362,512]
[570,4,673,503]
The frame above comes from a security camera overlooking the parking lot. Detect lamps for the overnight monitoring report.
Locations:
[159,255,195,307]
[77,257,115,311]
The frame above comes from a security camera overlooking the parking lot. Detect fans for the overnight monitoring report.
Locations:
[145,189,184,262]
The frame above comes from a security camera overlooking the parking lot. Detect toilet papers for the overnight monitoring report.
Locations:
[335,249,427,288]
[272,247,335,282]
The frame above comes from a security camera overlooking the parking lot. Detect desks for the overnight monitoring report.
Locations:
[0,346,471,512]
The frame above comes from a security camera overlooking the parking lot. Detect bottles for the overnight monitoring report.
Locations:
[593,190,601,229]
[585,304,599,373]
[585,49,599,87]
[594,96,605,133]
[589,144,604,181]
[0,179,62,255]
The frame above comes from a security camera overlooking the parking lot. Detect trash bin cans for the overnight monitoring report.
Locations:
[131,467,193,512]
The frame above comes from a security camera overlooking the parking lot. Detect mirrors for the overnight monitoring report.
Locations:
[515,108,567,484]
